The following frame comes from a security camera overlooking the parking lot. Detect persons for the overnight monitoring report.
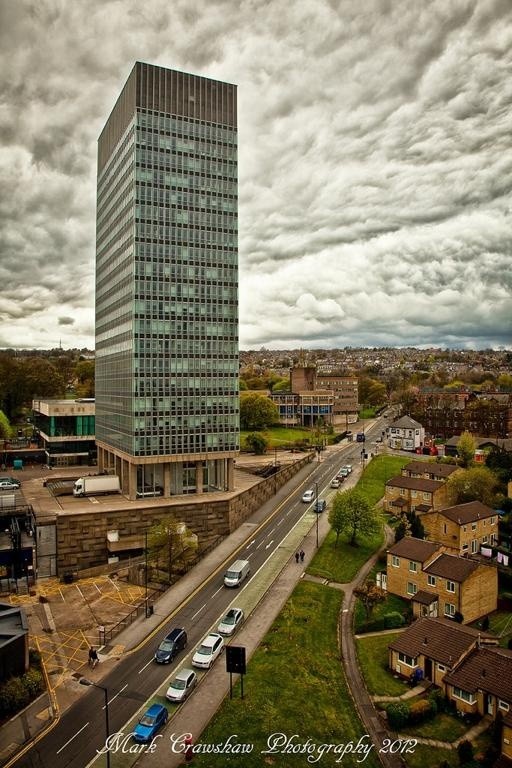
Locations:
[295,553,299,563]
[88,647,94,664]
[91,650,98,669]
[300,550,305,561]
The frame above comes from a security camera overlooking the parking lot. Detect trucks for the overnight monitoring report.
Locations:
[357,433,364,442]
[73,475,121,497]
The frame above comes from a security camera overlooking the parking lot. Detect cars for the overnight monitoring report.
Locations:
[166,669,197,703]
[331,465,352,488]
[0,482,19,490]
[133,704,168,745]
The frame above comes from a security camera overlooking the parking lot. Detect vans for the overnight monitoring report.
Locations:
[154,628,186,666]
[218,608,244,636]
[313,499,325,512]
[192,632,224,669]
[302,490,314,502]
[223,560,250,588]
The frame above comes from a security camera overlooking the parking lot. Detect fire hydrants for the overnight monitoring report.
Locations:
[182,735,194,761]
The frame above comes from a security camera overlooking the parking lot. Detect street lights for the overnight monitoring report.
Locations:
[80,679,110,767]
[304,481,318,548]
